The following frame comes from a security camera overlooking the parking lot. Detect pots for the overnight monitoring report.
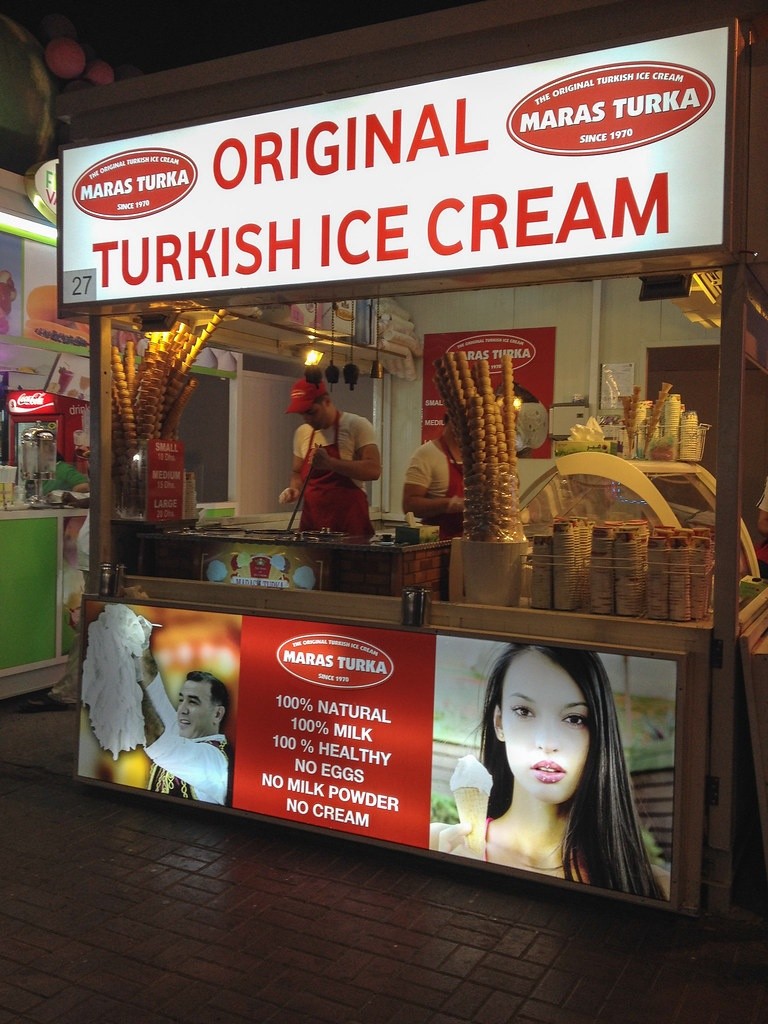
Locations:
[301,528,349,537]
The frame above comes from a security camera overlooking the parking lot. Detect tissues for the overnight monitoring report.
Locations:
[393,511,439,543]
[554,417,618,457]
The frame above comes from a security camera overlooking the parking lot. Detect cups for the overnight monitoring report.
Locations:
[183,472,198,519]
[619,395,702,460]
[530,516,716,621]
[0,483,14,510]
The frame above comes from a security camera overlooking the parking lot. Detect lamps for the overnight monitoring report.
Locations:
[304,348,325,367]
[493,381,539,411]
[637,274,694,302]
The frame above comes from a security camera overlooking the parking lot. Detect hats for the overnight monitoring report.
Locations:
[283,378,327,416]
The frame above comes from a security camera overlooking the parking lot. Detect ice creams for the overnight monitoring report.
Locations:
[450,756,493,856]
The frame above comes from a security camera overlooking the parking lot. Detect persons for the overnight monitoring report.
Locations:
[756,479,768,535]
[278,378,382,535]
[429,641,670,902]
[402,413,467,538]
[29,429,88,494]
[132,616,229,806]
